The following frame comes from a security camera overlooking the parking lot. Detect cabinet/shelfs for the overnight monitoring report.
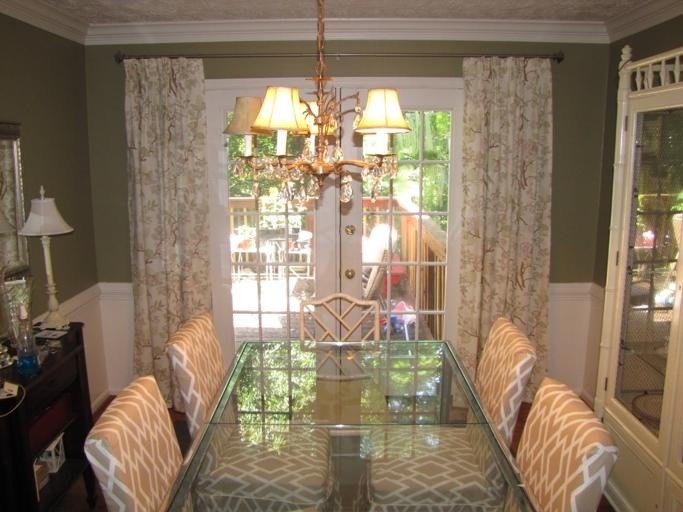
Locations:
[0,320,97,512]
[592,43,683,512]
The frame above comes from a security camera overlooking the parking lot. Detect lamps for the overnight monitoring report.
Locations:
[15,185,77,332]
[221,0,412,205]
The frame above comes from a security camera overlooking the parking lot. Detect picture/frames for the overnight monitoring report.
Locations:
[0,120,32,282]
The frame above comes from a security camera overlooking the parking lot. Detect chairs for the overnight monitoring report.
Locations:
[230,224,314,280]
[162,312,345,511]
[289,292,396,458]
[503,375,622,512]
[352,316,538,512]
[82,374,194,512]
[360,223,399,337]
